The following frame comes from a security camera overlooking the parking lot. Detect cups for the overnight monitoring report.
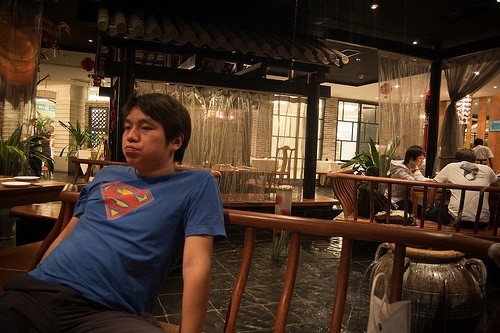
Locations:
[41,166,49,181]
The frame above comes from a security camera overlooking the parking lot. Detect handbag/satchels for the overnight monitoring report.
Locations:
[366,272,411,332]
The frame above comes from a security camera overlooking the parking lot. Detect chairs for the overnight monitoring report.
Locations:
[440,157,489,166]
[0,182,80,289]
[268,145,295,186]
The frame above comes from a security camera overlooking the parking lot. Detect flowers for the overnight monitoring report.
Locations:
[30,110,55,139]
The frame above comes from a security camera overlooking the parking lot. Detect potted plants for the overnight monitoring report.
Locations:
[58,119,103,177]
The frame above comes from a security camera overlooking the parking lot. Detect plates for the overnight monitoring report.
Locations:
[2,182,31,187]
[14,176,40,180]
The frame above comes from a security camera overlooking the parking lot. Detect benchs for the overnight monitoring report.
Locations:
[71,157,222,199]
[29,191,425,332]
[327,167,499,241]
[216,193,341,225]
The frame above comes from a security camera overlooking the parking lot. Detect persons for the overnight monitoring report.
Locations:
[383,145,434,220]
[0,93,226,333]
[471,138,497,173]
[424,147,500,229]
[26,118,55,158]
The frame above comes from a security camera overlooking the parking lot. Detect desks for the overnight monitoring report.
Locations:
[316,160,341,187]
[412,187,452,218]
[176,165,266,194]
[9,201,62,245]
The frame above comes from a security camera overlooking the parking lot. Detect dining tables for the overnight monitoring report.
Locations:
[0,174,65,190]
